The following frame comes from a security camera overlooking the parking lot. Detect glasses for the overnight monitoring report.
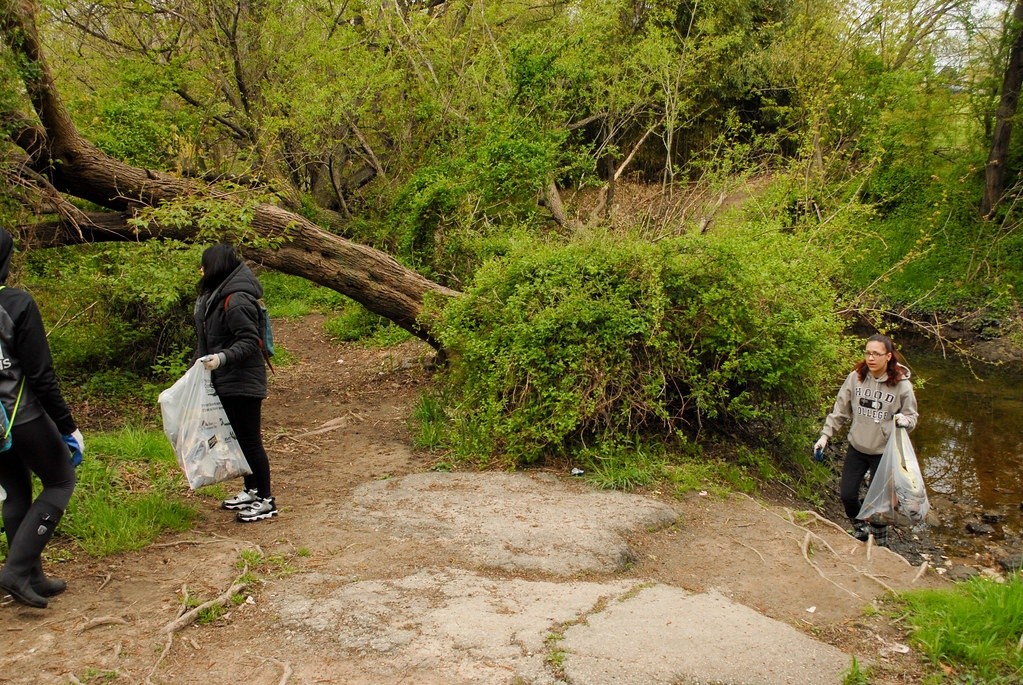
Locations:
[864,351,887,358]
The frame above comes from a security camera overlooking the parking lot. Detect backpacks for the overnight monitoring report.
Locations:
[224,292,275,360]
[0,286,26,452]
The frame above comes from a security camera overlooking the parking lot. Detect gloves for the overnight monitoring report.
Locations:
[814,435,829,462]
[62,427,86,467]
[894,413,909,428]
[201,353,221,370]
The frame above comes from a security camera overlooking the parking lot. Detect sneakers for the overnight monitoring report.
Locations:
[222,487,258,509]
[237,492,278,522]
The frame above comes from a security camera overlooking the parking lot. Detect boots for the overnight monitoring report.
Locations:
[854,519,869,539]
[2,499,66,598]
[870,523,889,550]
[0,498,65,608]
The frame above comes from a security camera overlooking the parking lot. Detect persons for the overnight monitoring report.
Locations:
[192,245,280,522]
[814,335,918,547]
[0,229,85,607]
